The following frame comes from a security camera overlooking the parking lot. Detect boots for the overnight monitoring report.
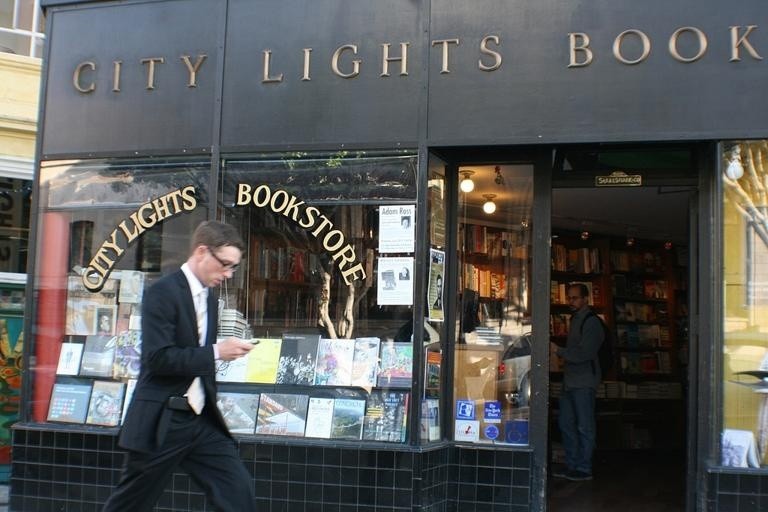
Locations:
[553,468,592,481]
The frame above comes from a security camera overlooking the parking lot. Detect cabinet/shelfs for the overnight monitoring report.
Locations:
[455,214,689,442]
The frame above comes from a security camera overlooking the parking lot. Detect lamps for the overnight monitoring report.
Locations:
[459,170,475,193]
[481,193,496,215]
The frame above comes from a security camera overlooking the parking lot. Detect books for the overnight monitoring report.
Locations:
[460,224,532,326]
[550,243,689,397]
[215,334,440,443]
[47,267,147,428]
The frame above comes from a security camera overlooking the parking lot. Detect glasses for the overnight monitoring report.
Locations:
[207,247,240,272]
[566,296,578,301]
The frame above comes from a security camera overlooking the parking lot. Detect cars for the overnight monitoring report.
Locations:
[425,331,533,404]
[0,272,28,314]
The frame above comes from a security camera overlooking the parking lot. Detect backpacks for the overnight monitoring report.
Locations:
[600,324,616,374]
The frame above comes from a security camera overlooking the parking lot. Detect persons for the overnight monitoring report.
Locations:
[101,219,255,511]
[550,283,604,482]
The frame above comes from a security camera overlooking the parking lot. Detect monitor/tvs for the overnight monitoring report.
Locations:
[481,301,504,319]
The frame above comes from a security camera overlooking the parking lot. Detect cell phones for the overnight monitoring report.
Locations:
[246,340,260,345]
